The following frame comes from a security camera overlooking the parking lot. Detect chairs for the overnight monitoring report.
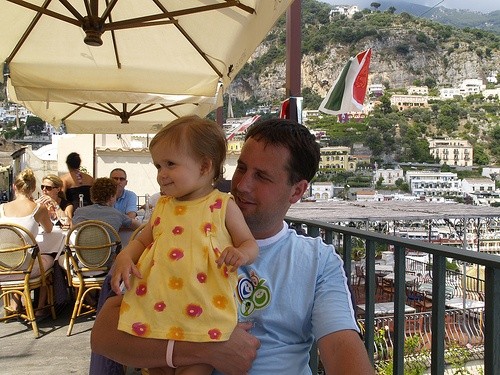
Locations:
[0,223,57,339]
[63,221,122,337]
[350,265,432,335]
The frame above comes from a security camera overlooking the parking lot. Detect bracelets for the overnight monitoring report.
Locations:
[166,340,177,369]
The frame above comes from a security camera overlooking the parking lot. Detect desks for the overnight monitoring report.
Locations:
[33,214,149,255]
[446,297,485,309]
[356,302,415,317]
[375,265,393,277]
[384,272,419,292]
[409,282,455,299]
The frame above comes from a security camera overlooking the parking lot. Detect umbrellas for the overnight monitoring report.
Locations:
[0,0,296,136]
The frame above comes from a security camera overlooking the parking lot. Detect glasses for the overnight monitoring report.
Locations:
[112,177,125,181]
[40,185,58,191]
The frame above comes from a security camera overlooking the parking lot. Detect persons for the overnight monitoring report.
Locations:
[91,118,377,375]
[110,114,258,375]
[109,168,138,218]
[61,152,94,211]
[36,173,74,226]
[0,170,54,318]
[71,177,142,310]
